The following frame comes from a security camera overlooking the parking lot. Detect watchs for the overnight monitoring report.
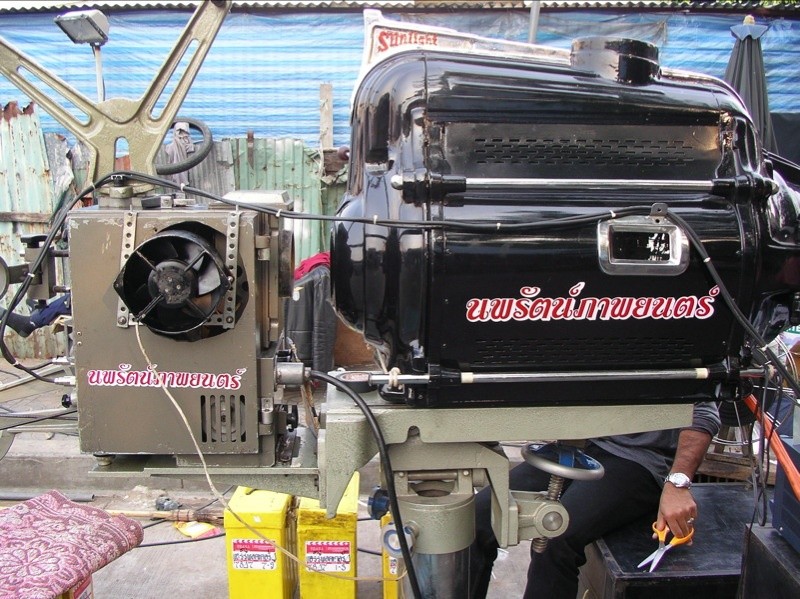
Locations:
[664,472,692,489]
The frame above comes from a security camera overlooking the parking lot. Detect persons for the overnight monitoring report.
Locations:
[473,400,722,599]
[1,293,73,338]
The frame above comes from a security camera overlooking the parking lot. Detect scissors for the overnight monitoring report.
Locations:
[636,521,695,573]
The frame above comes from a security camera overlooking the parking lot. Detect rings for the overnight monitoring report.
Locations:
[688,518,694,524]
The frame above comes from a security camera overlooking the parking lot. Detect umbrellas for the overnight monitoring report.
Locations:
[723,15,782,158]
